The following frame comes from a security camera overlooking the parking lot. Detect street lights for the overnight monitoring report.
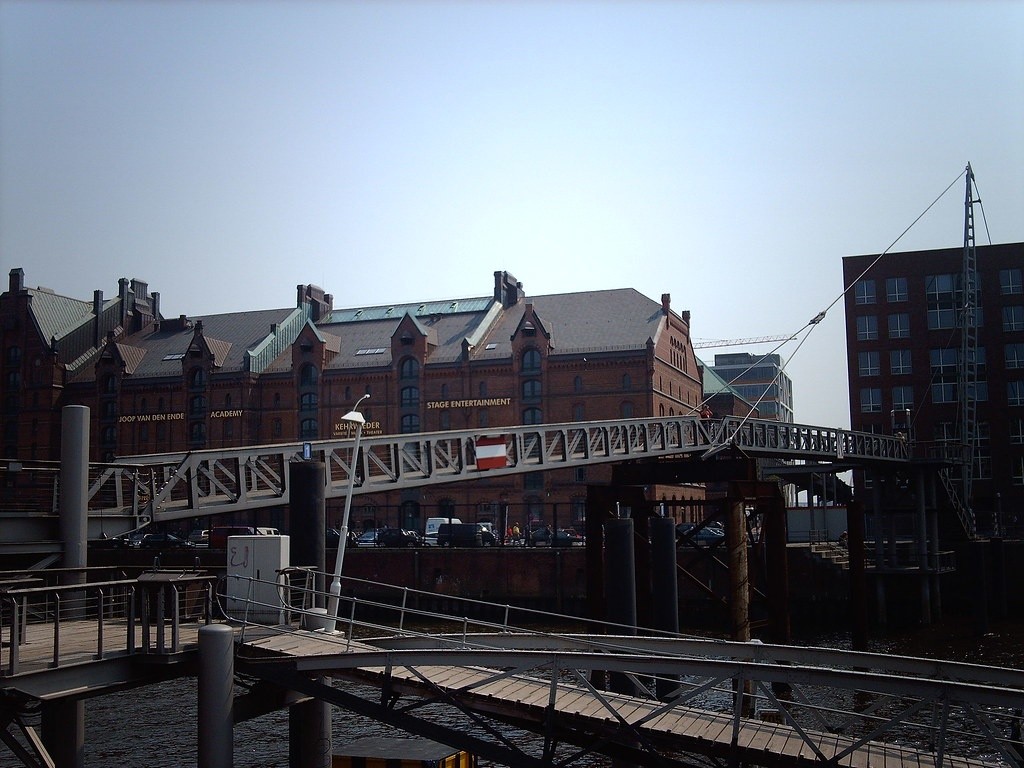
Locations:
[315,390,370,638]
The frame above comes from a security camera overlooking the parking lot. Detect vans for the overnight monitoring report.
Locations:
[326,523,355,548]
[209,525,281,549]
[380,526,425,548]
[435,523,501,547]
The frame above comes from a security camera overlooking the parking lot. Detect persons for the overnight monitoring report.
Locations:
[508,525,513,546]
[544,524,554,548]
[700,404,713,444]
[523,523,536,548]
[897,432,906,444]
[839,531,848,549]
[513,521,522,548]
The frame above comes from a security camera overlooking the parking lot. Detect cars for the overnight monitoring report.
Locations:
[140,533,196,549]
[681,526,726,547]
[357,525,380,547]
[527,526,572,547]
[90,536,133,548]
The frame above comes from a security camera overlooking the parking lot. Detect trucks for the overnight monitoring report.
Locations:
[424,517,464,546]
